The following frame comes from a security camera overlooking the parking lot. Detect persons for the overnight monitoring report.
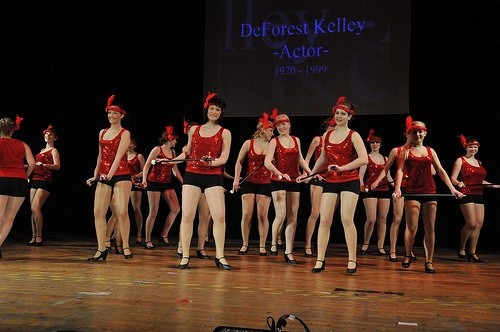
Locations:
[233,120,275,257]
[451,136,500,262]
[106,122,244,259]
[264,113,322,264]
[296,99,369,274]
[394,120,463,274]
[302,117,338,258]
[0,118,36,256]
[370,128,437,262]
[359,133,394,256]
[26,129,60,246]
[86,104,133,262]
[156,96,234,270]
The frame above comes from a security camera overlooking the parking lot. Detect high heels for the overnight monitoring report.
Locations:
[277,239,284,249]
[270,244,278,256]
[424,262,436,273]
[115,245,124,254]
[196,249,211,259]
[389,251,398,262]
[304,248,313,258]
[284,252,296,264]
[312,259,326,272]
[402,256,411,268]
[361,243,369,255]
[159,234,171,246]
[215,257,234,270]
[239,245,249,254]
[467,250,485,263]
[377,248,387,256]
[87,246,112,262]
[123,248,133,259]
[105,241,112,253]
[259,246,267,255]
[178,257,190,268]
[411,252,417,260]
[176,242,183,258]
[458,248,466,259]
[346,260,357,274]
[136,236,142,244]
[26,236,42,246]
[144,239,154,249]
